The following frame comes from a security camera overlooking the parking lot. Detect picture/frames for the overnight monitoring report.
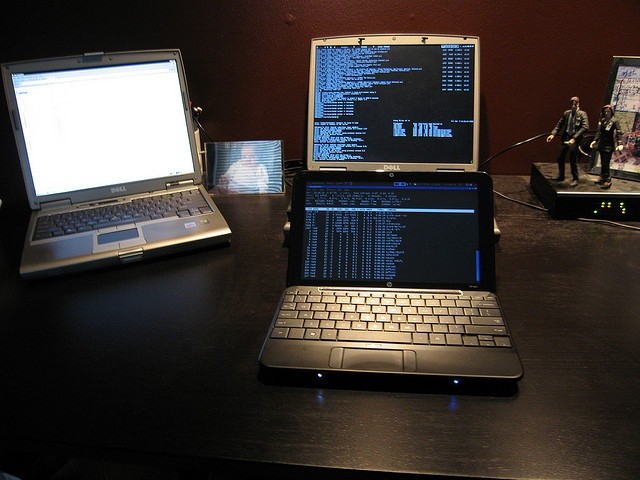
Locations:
[202,138,287,198]
[587,56,640,183]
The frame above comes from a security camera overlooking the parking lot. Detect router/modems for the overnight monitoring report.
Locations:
[530,162,640,221]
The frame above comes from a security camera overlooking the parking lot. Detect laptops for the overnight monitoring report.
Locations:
[256,170,525,382]
[0,48,233,275]
[284,33,500,239]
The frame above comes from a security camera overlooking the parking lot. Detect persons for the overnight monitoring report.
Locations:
[219,143,270,195]
[591,104,624,189]
[545,96,591,189]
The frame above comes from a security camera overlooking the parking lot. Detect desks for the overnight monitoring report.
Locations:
[2,175,638,480]
